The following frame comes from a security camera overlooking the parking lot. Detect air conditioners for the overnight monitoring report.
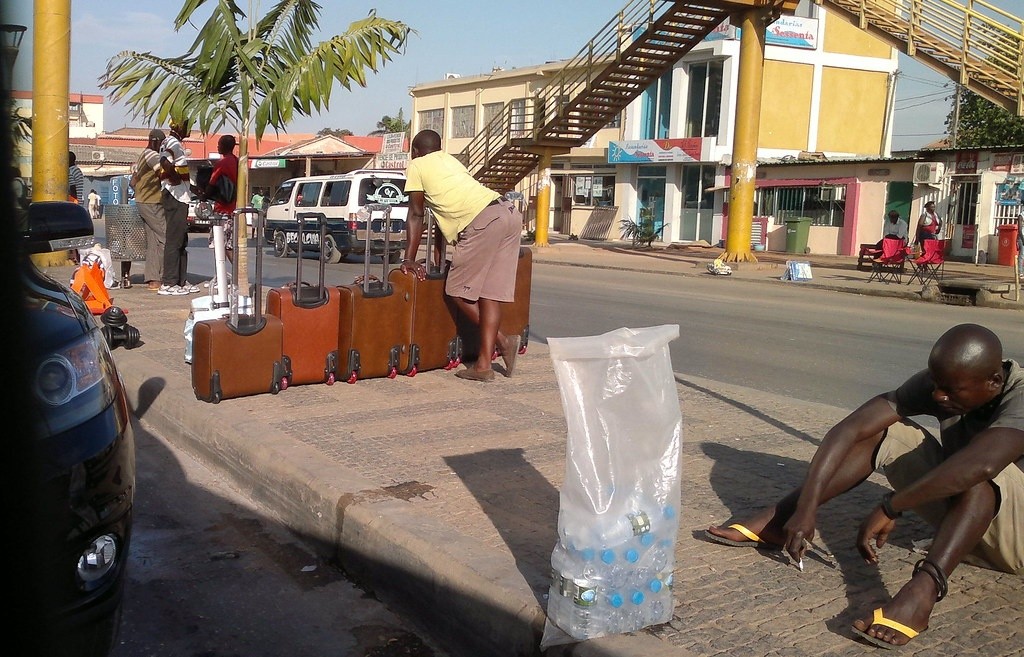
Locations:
[912,161,945,184]
[92,151,105,161]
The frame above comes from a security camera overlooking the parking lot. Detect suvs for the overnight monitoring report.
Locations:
[186,178,229,233]
[1,200,144,657]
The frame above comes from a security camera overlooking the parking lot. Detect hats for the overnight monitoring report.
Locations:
[168,115,197,129]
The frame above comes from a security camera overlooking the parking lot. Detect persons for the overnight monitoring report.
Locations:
[873,210,908,259]
[703,324,1024,649]
[13,166,28,209]
[87,189,101,219]
[129,129,208,295]
[1016,200,1024,284]
[297,192,303,205]
[251,188,265,240]
[401,130,522,382]
[914,201,942,272]
[205,133,239,288]
[67,151,84,264]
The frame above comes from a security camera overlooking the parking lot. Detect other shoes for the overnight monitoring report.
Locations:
[148,281,162,290]
[203,271,233,289]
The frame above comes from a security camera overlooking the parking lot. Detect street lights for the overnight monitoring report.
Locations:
[0,21,29,200]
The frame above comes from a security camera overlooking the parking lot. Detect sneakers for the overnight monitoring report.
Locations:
[456,362,495,382]
[157,278,200,296]
[499,335,521,377]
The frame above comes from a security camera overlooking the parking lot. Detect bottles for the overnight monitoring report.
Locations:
[546,504,676,640]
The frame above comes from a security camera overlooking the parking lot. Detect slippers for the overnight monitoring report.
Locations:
[851,606,929,650]
[704,523,783,551]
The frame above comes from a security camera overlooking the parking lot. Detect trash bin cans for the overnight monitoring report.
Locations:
[995,223,1019,266]
[785,215,810,254]
[104,203,147,261]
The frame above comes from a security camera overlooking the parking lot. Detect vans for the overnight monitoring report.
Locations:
[263,167,409,263]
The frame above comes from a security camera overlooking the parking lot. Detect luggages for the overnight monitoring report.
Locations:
[388,206,457,378]
[191,208,283,404]
[457,192,532,361]
[265,213,340,389]
[336,202,400,384]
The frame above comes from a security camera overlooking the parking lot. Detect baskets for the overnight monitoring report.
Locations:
[103,204,147,262]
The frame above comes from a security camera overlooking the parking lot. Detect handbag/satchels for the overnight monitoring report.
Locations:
[196,166,234,203]
[79,243,116,290]
[183,271,254,363]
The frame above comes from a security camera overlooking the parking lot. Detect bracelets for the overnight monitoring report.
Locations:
[881,490,902,519]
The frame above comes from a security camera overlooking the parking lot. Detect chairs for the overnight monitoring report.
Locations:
[904,239,951,286]
[867,239,910,284]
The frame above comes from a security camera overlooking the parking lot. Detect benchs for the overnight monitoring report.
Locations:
[858,244,908,273]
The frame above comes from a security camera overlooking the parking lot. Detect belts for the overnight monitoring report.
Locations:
[486,196,510,206]
